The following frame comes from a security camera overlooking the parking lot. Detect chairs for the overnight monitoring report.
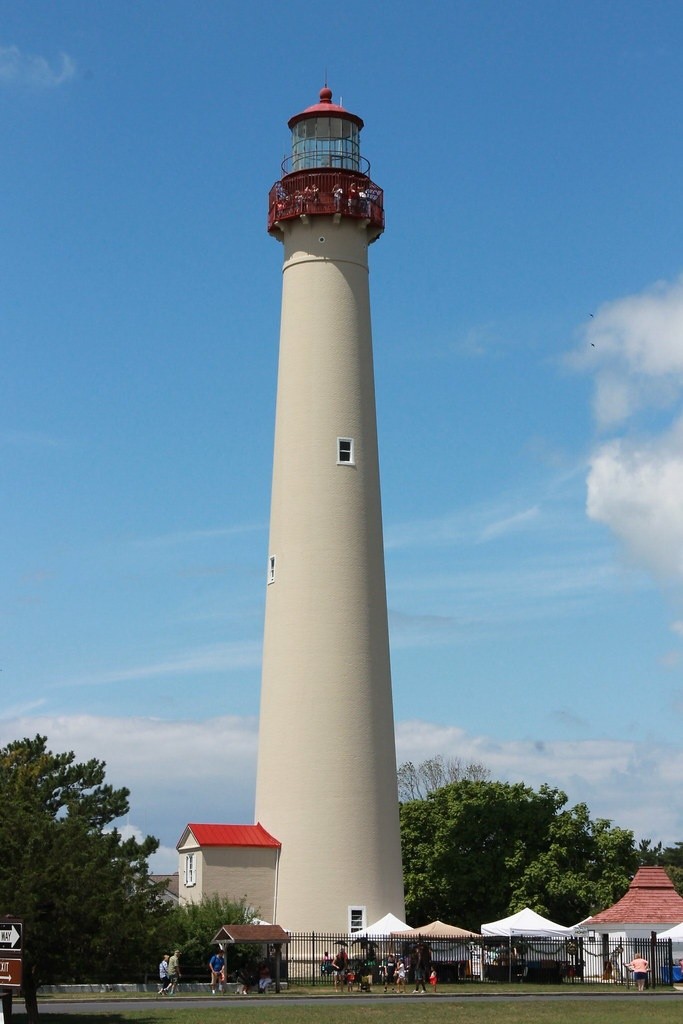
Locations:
[517,967,528,982]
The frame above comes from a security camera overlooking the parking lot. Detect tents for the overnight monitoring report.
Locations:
[649,922,683,943]
[348,908,592,941]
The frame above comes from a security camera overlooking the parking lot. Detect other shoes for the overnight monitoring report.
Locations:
[212,989,216,995]
[158,989,167,996]
[403,991,405,994]
[170,990,176,997]
[384,989,388,993]
[421,990,427,994]
[397,990,400,994]
[434,990,436,993]
[413,990,419,994]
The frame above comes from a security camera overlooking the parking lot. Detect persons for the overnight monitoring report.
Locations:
[624,954,648,992]
[304,184,361,214]
[321,944,437,994]
[238,960,274,995]
[209,950,225,994]
[158,950,182,996]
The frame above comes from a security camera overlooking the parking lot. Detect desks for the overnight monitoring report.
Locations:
[487,965,560,984]
[662,966,683,984]
